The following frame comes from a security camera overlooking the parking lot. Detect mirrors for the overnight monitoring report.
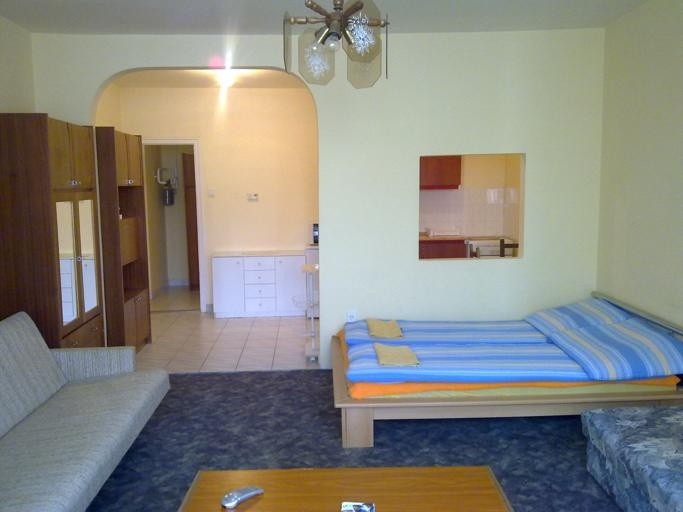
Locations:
[55,198,100,329]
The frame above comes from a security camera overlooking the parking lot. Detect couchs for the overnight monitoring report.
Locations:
[0,308,172,512]
[577,399,683,512]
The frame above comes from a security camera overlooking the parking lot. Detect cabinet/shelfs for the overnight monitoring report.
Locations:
[97,127,153,356]
[210,253,321,320]
[0,113,107,357]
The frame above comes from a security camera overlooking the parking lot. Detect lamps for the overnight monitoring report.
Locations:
[284,0,391,69]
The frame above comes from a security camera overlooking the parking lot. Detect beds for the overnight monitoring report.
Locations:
[329,289,683,452]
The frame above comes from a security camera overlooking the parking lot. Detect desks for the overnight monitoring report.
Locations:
[174,463,516,512]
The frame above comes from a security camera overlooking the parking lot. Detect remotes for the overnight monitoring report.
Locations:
[221,486,264,509]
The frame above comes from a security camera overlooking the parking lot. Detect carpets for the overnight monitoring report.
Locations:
[91,367,634,512]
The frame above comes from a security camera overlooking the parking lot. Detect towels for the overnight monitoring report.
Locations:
[364,318,421,369]
